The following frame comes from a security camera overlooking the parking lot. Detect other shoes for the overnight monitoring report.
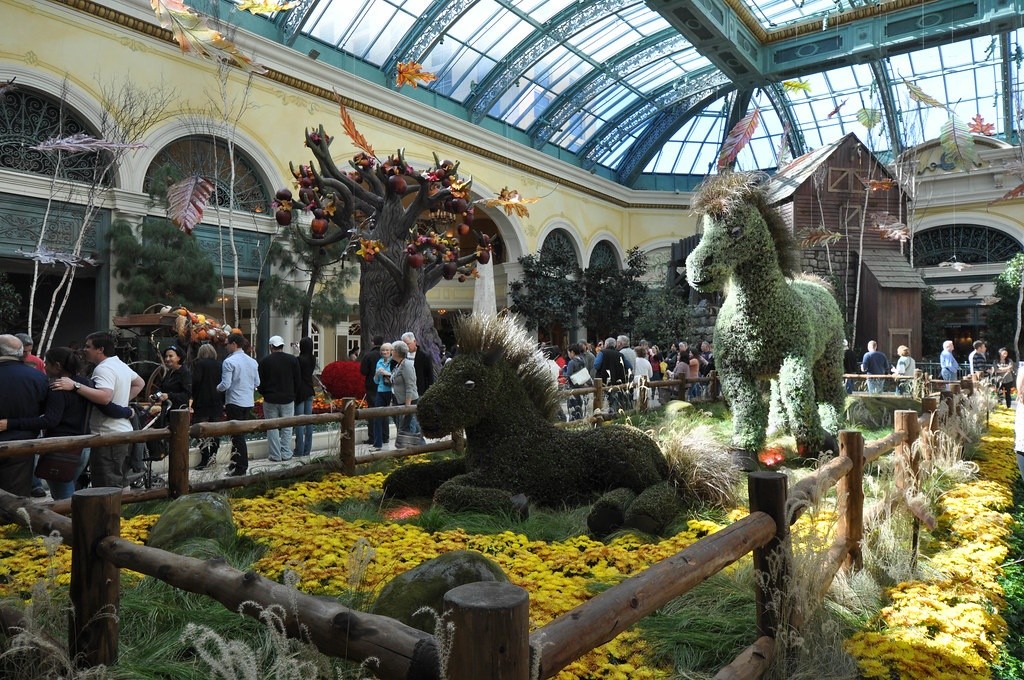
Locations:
[362,439,374,444]
[268,456,281,461]
[292,452,310,457]
[195,462,211,469]
[382,439,390,444]
[368,446,381,452]
[226,469,245,476]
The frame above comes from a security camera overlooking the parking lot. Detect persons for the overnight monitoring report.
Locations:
[939,340,961,389]
[969,341,987,375]
[539,336,720,423]
[842,340,857,395]
[216,333,261,476]
[992,347,1013,408]
[0,329,192,526]
[350,331,457,452]
[257,335,302,462]
[1013,365,1024,479]
[890,345,915,396]
[861,341,889,395]
[293,337,316,457]
[188,344,225,470]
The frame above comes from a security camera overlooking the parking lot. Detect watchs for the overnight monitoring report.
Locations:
[73,382,81,392]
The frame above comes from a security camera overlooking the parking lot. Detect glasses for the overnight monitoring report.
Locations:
[166,346,181,353]
[224,342,233,347]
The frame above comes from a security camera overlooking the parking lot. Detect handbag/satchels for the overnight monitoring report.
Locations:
[570,359,591,387]
[395,430,422,448]
[382,375,392,386]
[34,451,82,483]
[659,361,668,373]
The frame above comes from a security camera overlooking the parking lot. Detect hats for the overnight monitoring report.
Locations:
[268,335,285,347]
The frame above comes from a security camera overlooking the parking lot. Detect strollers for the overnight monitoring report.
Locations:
[75,396,172,492]
